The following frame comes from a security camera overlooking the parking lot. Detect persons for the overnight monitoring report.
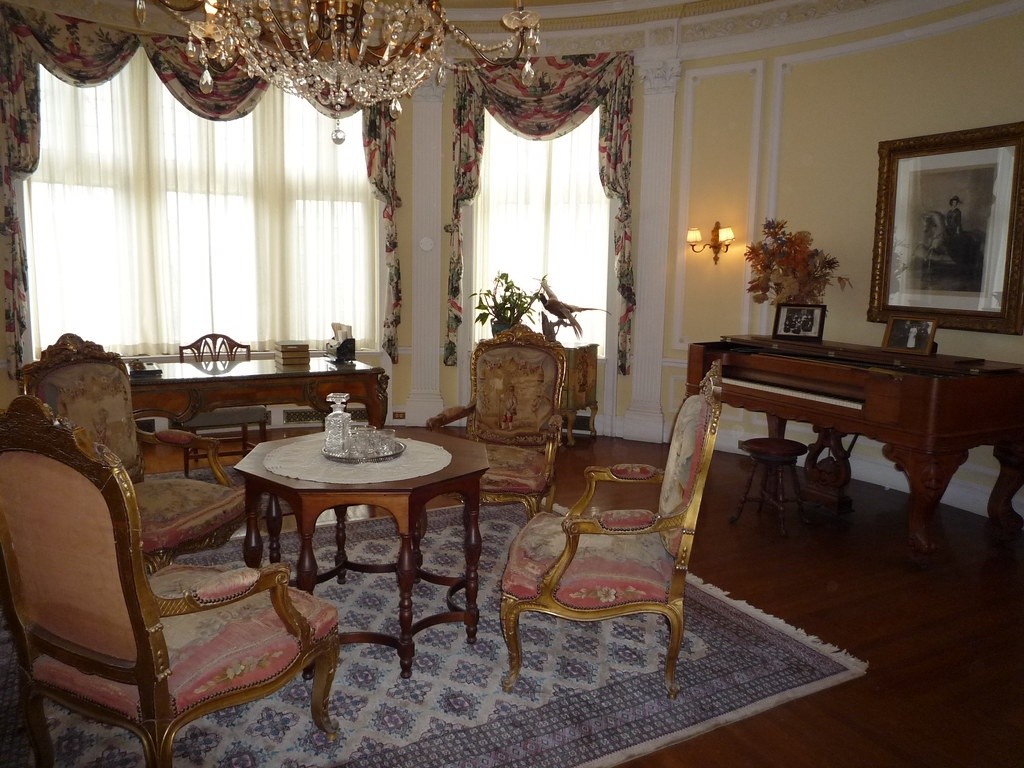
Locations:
[917,323,928,348]
[907,324,917,348]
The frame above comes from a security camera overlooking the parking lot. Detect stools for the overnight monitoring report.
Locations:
[729,437,809,536]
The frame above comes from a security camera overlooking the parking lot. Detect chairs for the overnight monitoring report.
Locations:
[501,360,725,693]
[0,397,341,767]
[427,324,568,523]
[178,334,270,476]
[17,334,249,573]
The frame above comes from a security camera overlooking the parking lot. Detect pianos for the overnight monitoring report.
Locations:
[682,335,1024,566]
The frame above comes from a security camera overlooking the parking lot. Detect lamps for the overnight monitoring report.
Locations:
[687,220,734,261]
[137,1,543,146]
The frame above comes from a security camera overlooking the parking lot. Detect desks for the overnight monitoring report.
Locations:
[234,430,488,680]
[482,338,603,446]
[126,356,389,475]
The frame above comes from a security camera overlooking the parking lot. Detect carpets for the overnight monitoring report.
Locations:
[0,497,871,768]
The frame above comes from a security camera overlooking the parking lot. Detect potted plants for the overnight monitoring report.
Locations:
[464,276,545,338]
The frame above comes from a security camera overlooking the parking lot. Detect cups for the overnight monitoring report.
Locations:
[349,421,396,457]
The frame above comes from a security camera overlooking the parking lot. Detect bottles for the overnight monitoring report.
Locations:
[324,392,351,457]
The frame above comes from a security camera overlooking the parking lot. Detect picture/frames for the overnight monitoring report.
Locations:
[772,302,829,342]
[882,317,936,356]
[867,122,1023,335]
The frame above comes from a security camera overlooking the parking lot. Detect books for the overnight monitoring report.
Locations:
[274,342,310,365]
